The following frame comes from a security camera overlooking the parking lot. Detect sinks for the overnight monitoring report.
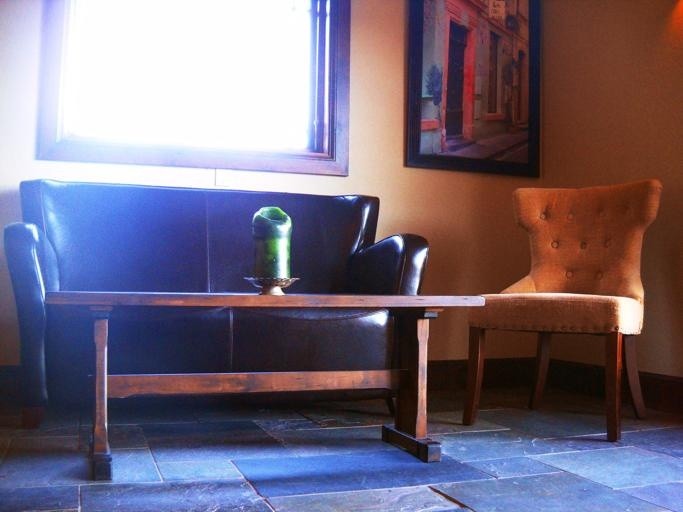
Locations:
[1,180,429,408]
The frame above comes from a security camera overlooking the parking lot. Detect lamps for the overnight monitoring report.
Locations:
[458,180,662,440]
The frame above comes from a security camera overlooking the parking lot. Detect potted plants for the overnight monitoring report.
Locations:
[404,1,539,178]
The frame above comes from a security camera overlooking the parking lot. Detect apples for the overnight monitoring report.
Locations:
[242,204,300,294]
